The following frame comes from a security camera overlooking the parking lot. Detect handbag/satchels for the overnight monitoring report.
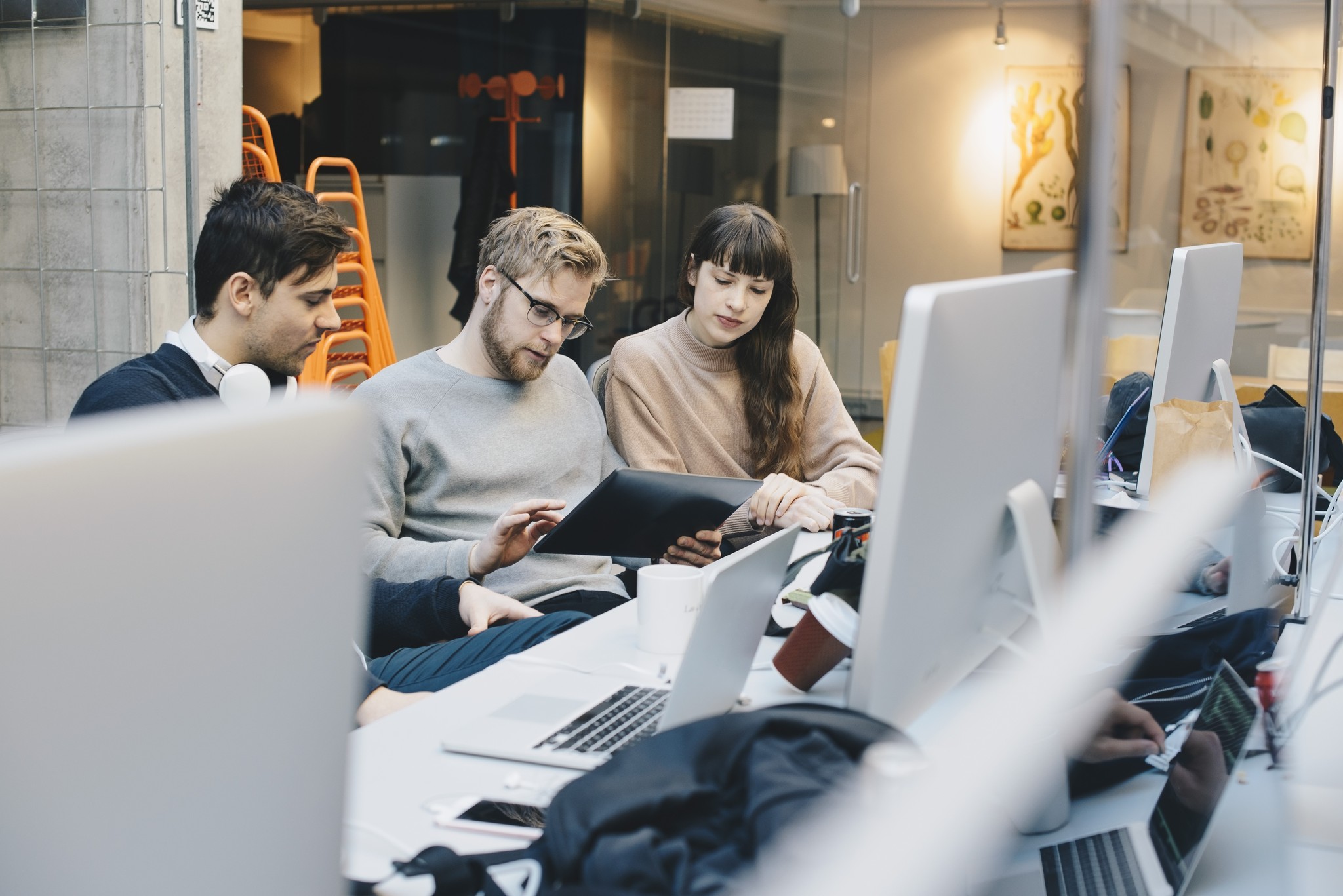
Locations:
[1107,372,1343,494]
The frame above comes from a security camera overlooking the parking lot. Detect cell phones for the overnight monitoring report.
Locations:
[435,796,546,841]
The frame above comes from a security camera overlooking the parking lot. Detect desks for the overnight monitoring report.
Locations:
[342,477,1317,896]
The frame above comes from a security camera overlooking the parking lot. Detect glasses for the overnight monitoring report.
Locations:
[500,268,594,340]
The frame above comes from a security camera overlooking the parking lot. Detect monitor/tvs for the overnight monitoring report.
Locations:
[843,270,1067,839]
[1133,244,1275,625]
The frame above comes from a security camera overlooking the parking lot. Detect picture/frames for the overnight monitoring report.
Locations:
[1179,65,1324,262]
[1000,61,1135,255]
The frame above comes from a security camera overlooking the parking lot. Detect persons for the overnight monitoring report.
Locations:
[605,203,882,533]
[1169,729,1228,822]
[66,174,594,729]
[1065,689,1165,763]
[347,207,722,617]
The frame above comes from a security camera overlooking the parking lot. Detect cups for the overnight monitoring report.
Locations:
[772,592,860,692]
[636,562,703,652]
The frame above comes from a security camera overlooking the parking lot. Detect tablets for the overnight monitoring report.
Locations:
[532,468,763,558]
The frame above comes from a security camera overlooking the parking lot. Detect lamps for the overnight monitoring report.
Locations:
[785,141,848,346]
[657,135,716,301]
[993,5,1007,45]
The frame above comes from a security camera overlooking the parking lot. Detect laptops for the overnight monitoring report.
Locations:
[0,387,373,896]
[443,522,800,768]
[1006,652,1264,896]
